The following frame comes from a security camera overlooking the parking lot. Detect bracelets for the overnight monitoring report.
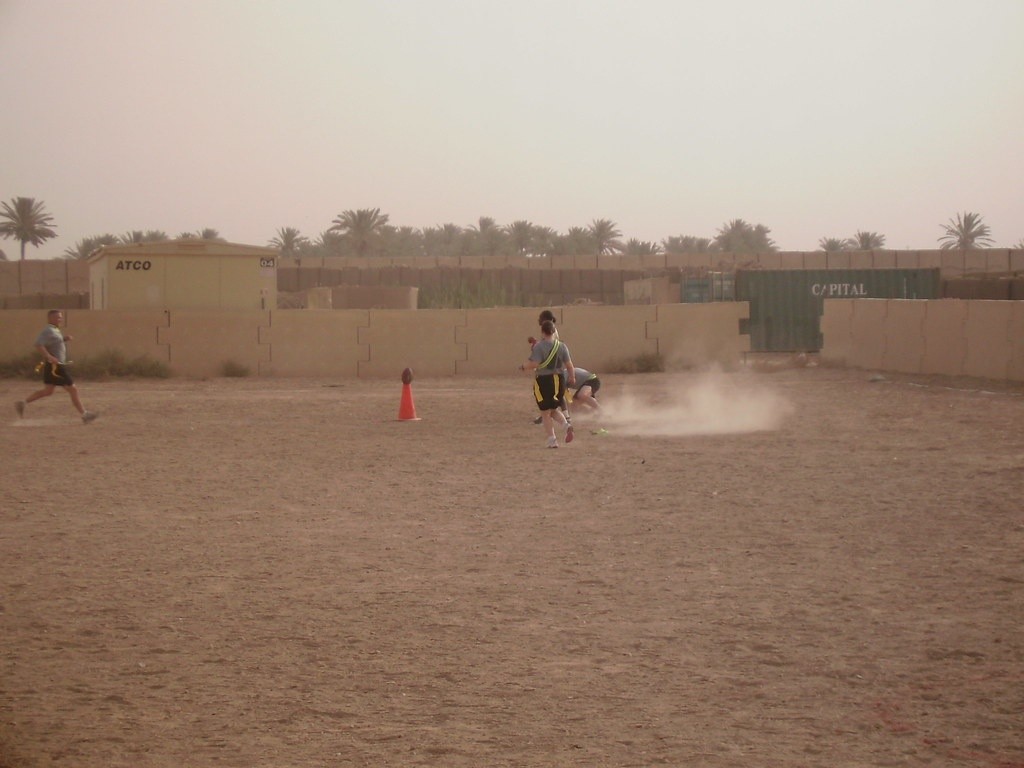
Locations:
[521,363,523,371]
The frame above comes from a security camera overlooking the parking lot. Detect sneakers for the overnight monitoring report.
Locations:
[564,424,574,443]
[544,437,558,448]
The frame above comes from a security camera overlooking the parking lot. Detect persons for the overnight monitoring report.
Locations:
[517,319,575,449]
[528,310,571,423]
[564,368,609,420]
[15,309,100,423]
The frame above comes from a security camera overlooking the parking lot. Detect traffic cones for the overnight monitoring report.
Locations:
[392,383,423,421]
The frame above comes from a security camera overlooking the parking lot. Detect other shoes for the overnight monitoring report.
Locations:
[81,411,100,425]
[534,415,542,424]
[13,401,25,419]
[565,416,571,423]
